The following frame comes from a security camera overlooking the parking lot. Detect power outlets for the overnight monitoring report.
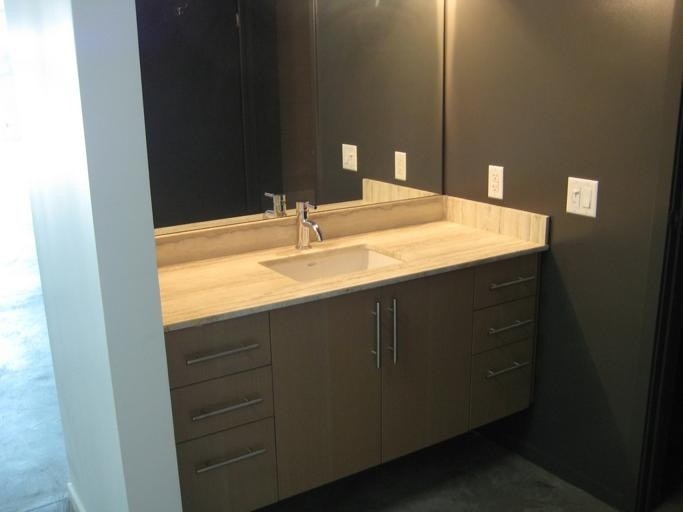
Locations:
[486,164,506,200]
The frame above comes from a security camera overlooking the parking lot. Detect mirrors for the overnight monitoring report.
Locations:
[134,1,445,266]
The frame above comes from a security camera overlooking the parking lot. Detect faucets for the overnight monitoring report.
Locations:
[263,193,288,221]
[295,202,322,250]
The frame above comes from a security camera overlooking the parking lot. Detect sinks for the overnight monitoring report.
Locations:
[258,244,409,283]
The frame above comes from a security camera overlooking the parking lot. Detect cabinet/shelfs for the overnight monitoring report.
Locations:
[166,253,277,512]
[266,253,473,499]
[440,195,548,431]
